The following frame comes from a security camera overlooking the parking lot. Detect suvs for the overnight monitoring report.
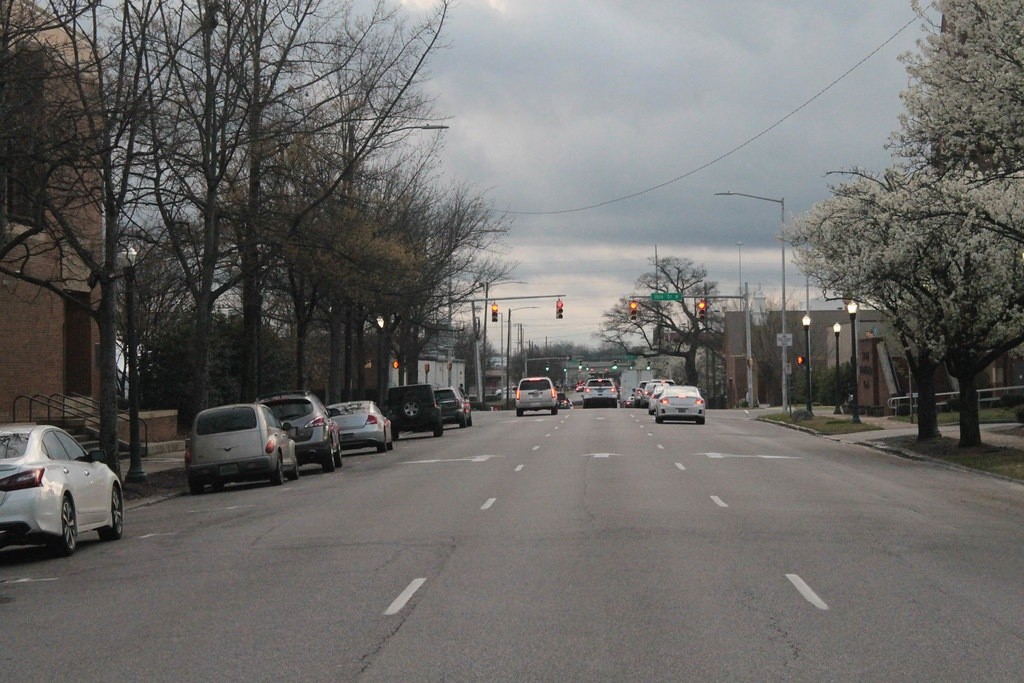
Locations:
[252,391,344,472]
[433,385,473,428]
[512,377,559,416]
[383,384,443,441]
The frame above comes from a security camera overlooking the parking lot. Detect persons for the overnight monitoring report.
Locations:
[459,383,465,394]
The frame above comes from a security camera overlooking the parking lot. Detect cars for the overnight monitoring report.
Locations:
[496,386,518,396]
[558,377,707,425]
[185,404,299,493]
[326,400,395,453]
[0,423,124,557]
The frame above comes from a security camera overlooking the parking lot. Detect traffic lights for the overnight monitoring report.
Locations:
[629,300,637,320]
[698,302,705,320]
[798,356,805,364]
[556,301,563,319]
[491,304,498,323]
[393,361,399,369]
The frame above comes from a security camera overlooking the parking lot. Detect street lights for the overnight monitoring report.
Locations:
[713,190,788,411]
[482,281,531,406]
[505,307,543,410]
[803,300,863,423]
[119,246,147,478]
